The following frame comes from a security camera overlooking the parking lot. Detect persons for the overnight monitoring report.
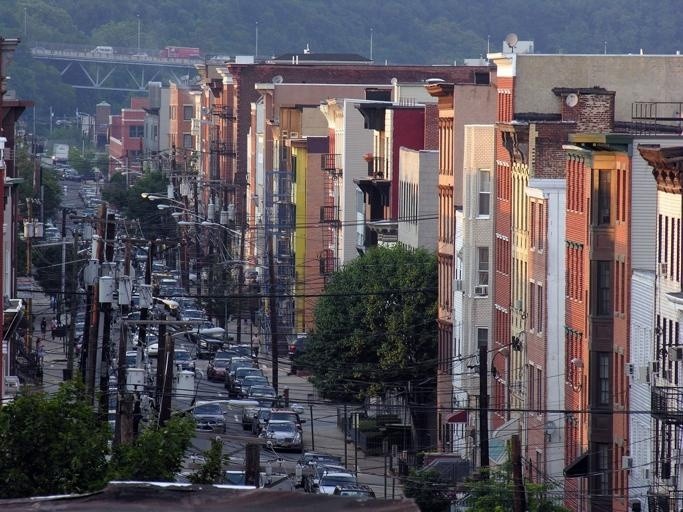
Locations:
[20,333,25,348]
[40,317,46,339]
[35,336,44,364]
[51,317,57,328]
[251,332,261,356]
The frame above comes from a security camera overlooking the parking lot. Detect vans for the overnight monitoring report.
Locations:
[91,46,113,54]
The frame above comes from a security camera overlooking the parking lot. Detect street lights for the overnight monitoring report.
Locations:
[369,27,373,60]
[24,7,26,39]
[605,40,607,54]
[139,189,279,390]
[136,14,140,49]
[488,34,490,65]
[49,106,54,134]
[255,20,259,57]
[75,108,79,127]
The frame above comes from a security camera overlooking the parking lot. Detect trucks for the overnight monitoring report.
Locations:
[159,46,199,60]
[52,144,69,164]
